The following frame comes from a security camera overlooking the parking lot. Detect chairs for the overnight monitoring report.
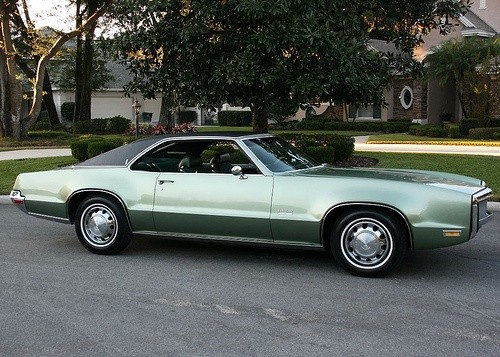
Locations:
[136,151,239,173]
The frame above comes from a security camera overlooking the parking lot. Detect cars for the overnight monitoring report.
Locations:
[10,131,493,281]
